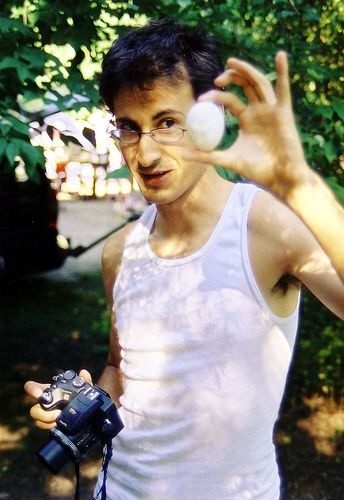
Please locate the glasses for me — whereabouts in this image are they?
[105,112,190,145]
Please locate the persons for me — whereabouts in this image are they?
[23,17,343,500]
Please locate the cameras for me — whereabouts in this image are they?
[37,369,124,476]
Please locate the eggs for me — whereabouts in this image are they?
[185,100,225,150]
